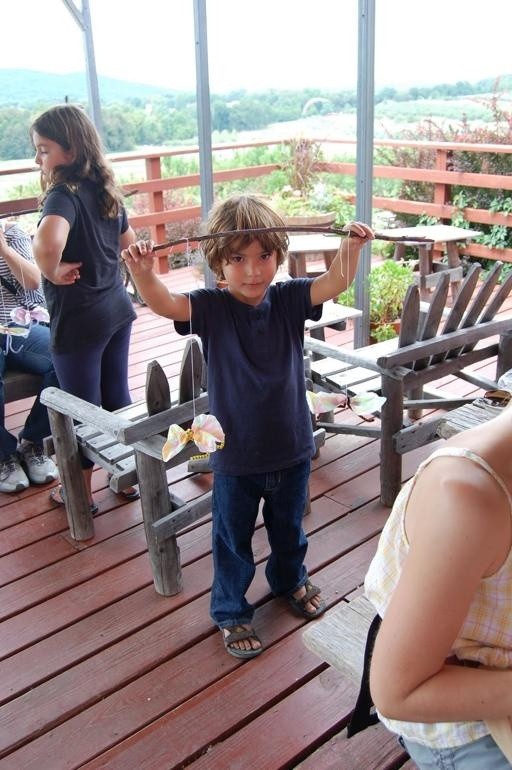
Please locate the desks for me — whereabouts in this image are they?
[376,223,483,307]
[282,232,342,304]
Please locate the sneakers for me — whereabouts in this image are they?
[0,452,30,493]
[17,431,60,486]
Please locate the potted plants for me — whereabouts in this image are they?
[333,259,417,345]
[267,133,350,237]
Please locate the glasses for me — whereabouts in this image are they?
[472,388,512,412]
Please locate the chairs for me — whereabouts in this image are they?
[305,263,512,515]
[42,333,328,597]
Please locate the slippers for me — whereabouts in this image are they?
[107,471,141,501]
[50,485,99,516]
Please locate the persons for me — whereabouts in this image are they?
[358,395,512,770]
[1,218,62,498]
[121,196,374,658]
[28,102,143,514]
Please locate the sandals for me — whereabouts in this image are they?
[283,575,328,619]
[219,617,264,660]
